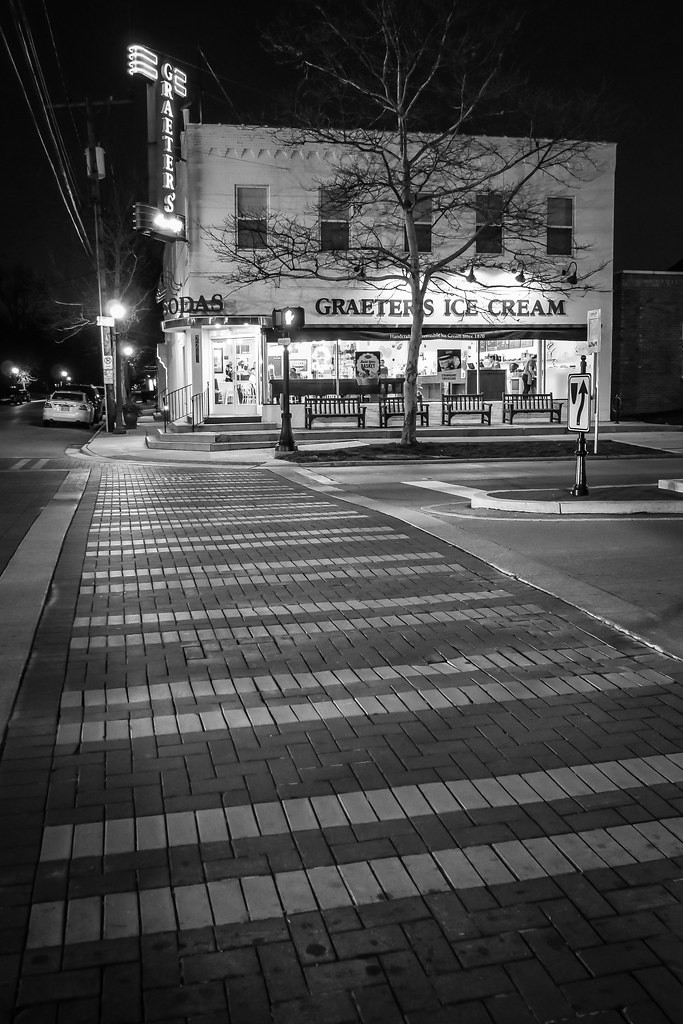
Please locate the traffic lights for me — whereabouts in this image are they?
[272,305,305,331]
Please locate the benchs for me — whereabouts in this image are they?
[378,394,430,428]
[502,392,564,425]
[441,391,493,427]
[305,396,367,430]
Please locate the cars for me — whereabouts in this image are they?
[43,383,105,428]
[0,386,31,405]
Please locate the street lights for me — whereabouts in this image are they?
[105,299,126,435]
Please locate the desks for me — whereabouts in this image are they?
[443,380,465,395]
[269,378,406,403]
[222,382,256,404]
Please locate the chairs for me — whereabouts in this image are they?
[219,383,257,405]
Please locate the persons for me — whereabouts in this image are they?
[522,355,538,400]
[487,356,499,368]
[380,360,388,378]
[290,368,297,378]
[225,365,233,382]
[249,362,257,404]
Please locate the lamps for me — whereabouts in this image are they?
[561,262,578,283]
[354,255,367,278]
[459,262,476,282]
[512,259,525,283]
[156,270,181,303]
